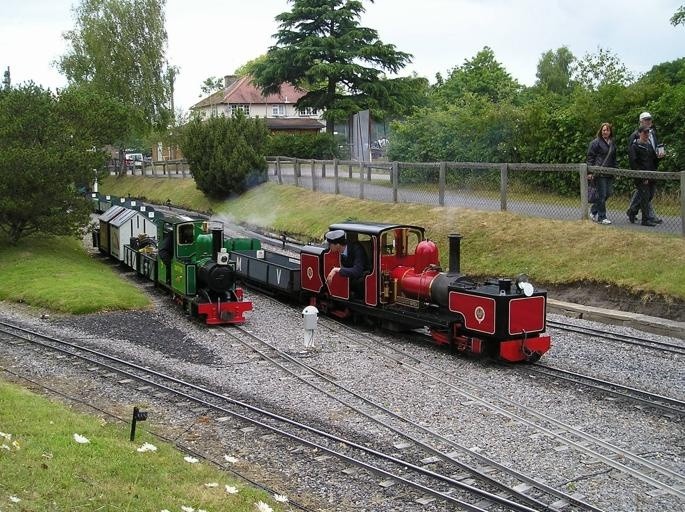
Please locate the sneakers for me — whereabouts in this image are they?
[627,209,663,226]
[598,219,612,225]
[590,211,596,221]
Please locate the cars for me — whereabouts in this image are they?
[126,154,143,169]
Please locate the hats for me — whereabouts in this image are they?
[321,230,345,248]
[640,112,651,118]
[638,126,650,132]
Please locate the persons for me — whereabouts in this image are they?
[629,113,669,224]
[627,124,658,227]
[322,227,373,301]
[586,123,617,224]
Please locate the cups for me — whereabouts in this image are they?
[657,144,665,155]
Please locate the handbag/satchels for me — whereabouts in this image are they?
[588,177,599,203]
